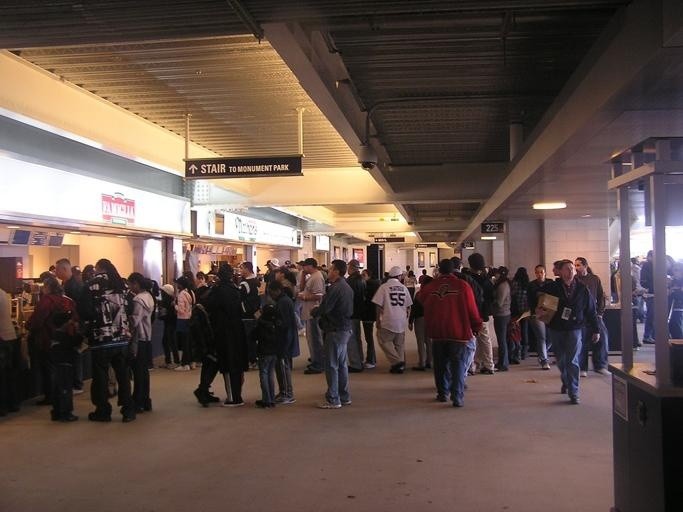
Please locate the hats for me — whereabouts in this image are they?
[241,255,408,278]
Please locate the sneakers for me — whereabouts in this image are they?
[3,384,296,422]
[579,370,588,378]
[450,396,465,408]
[595,367,612,376]
[561,384,568,393]
[316,397,351,408]
[436,393,449,400]
[570,394,580,405]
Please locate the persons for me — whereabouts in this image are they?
[1,249,683,423]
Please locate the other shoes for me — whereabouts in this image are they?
[390,361,405,373]
[542,359,549,369]
[426,365,432,368]
[249,363,260,369]
[304,369,320,374]
[510,359,520,365]
[364,362,375,368]
[480,368,494,374]
[413,366,424,371]
[496,366,509,371]
[348,366,363,373]
[161,361,201,371]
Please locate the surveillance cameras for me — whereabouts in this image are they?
[357,146,378,170]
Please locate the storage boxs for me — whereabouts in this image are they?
[537,293,559,325]
